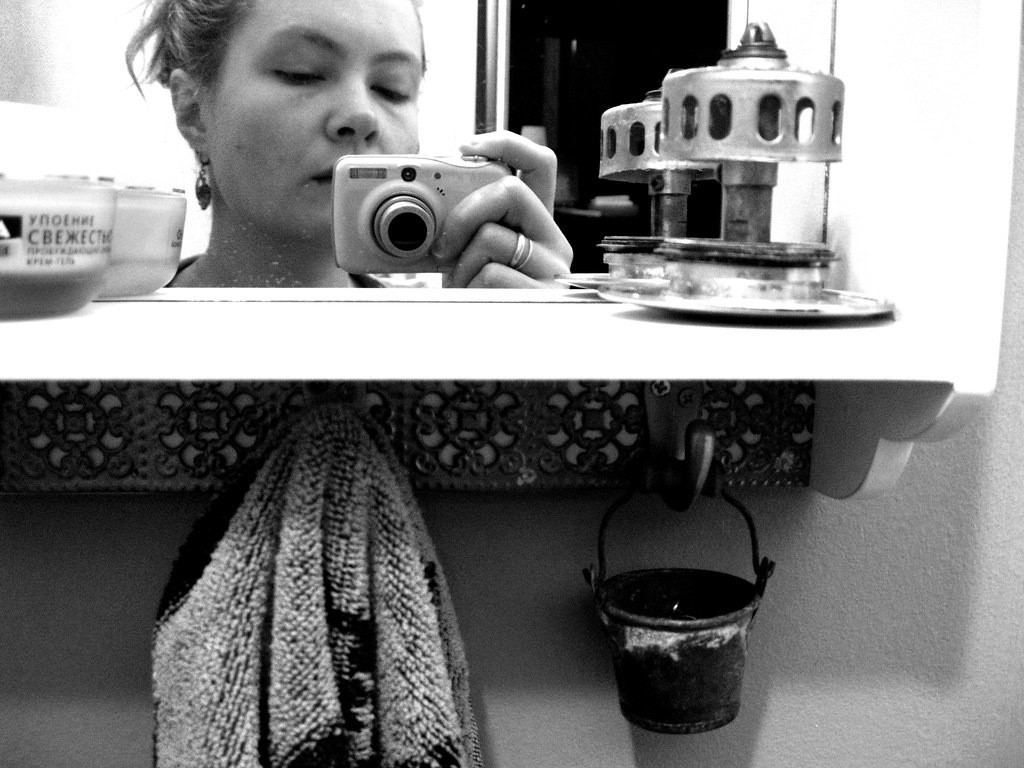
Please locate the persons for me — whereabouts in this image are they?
[125,0,573,288]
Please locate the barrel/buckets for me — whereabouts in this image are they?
[583,487,775,734]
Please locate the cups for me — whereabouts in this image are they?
[0,178,115,317]
[98,189,188,293]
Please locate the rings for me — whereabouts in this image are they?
[510,232,533,269]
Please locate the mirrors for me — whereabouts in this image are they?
[0,0,841,306]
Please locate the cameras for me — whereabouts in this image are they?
[331,154,514,275]
